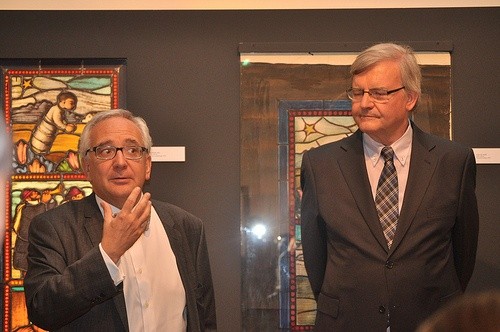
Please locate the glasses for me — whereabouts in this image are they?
[84,145,149,161]
[346,86,405,102]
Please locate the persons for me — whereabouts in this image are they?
[22,109,218,332]
[300,43,478,331]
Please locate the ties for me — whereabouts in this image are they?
[375,146,400,251]
